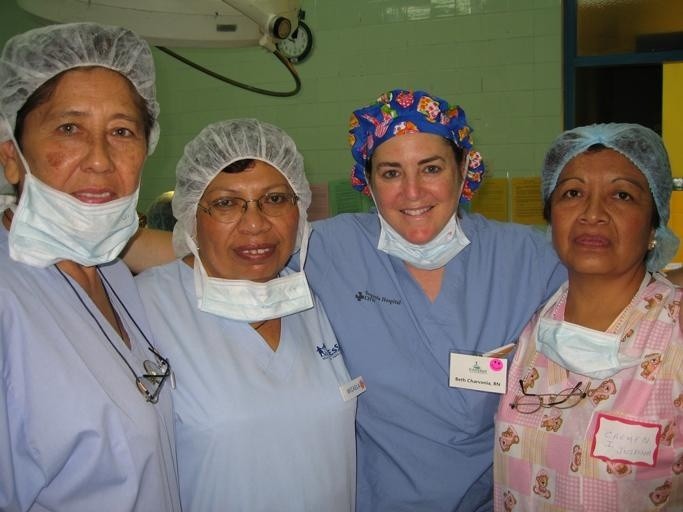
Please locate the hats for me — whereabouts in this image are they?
[348,90,484,204]
[541,123,679,273]
[0,22,161,156]
[171,118,312,258]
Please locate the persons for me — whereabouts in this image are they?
[491,123,682,512]
[116,89,568,511]
[132,117,365,511]
[1,20,179,512]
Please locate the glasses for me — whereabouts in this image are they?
[136,347,170,403]
[198,192,299,223]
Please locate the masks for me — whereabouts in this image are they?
[535,317,620,380]
[8,122,139,267]
[183,226,313,324]
[363,155,469,270]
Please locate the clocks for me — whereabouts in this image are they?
[275,20,312,62]
